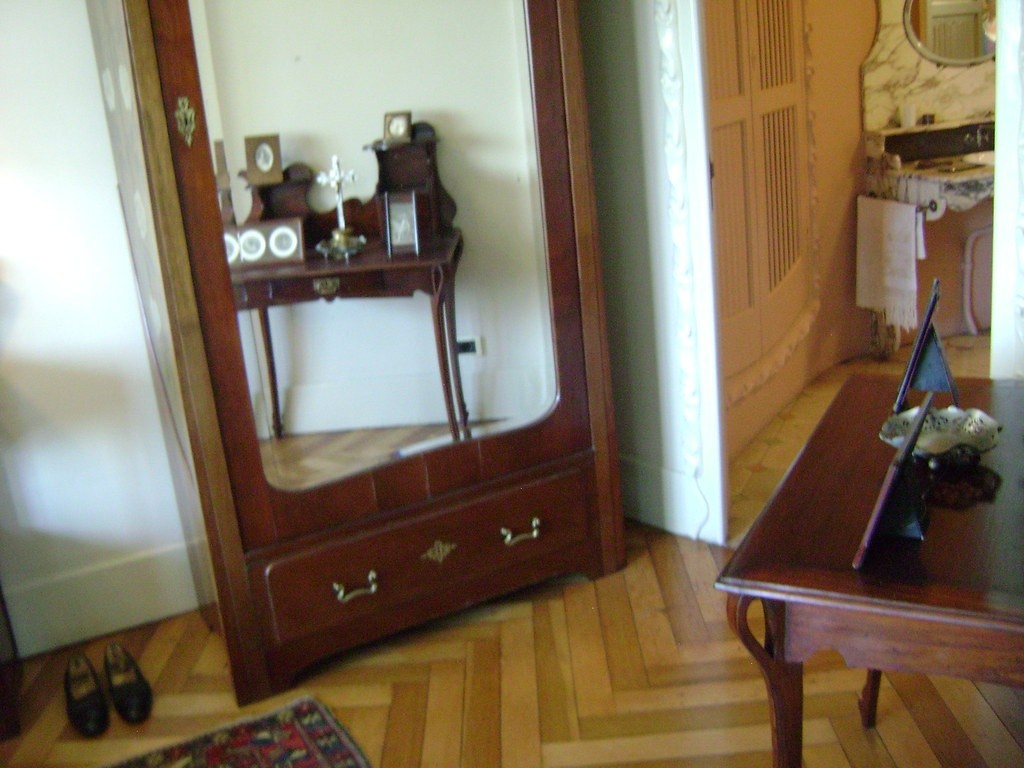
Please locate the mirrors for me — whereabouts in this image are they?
[903,1,997,68]
[176,1,563,491]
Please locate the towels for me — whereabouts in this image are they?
[856,194,926,334]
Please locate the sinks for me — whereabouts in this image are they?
[962,150,994,168]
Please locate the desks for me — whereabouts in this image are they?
[715,371,1024,767]
[226,230,474,457]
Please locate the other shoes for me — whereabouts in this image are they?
[63,652,108,737]
[103,644,152,725]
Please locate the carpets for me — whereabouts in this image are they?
[108,693,368,767]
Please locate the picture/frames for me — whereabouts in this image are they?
[382,110,412,144]
[245,134,283,187]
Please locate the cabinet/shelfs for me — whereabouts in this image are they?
[83,0,629,707]
